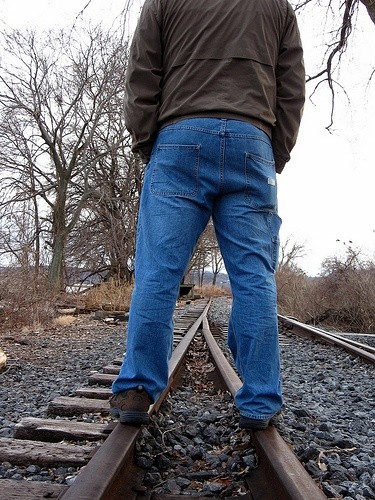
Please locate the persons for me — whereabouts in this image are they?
[105,0,305,432]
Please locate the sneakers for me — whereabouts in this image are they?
[109,387,154,424]
[239,413,272,430]
